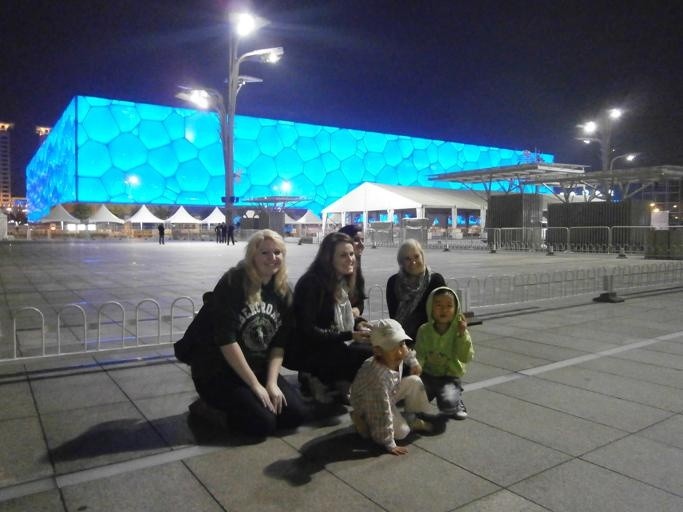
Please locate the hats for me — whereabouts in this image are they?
[370,319,413,351]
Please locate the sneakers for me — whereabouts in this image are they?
[455,400,467,419]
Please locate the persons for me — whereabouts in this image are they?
[227,222,234,246]
[387,239,447,376]
[338,225,373,333]
[158,223,164,245]
[349,319,434,456]
[412,286,474,420]
[221,222,227,243]
[173,229,308,436]
[281,233,374,405]
[215,223,221,243]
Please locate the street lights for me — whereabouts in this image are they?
[179,10,284,241]
[574,103,638,204]
